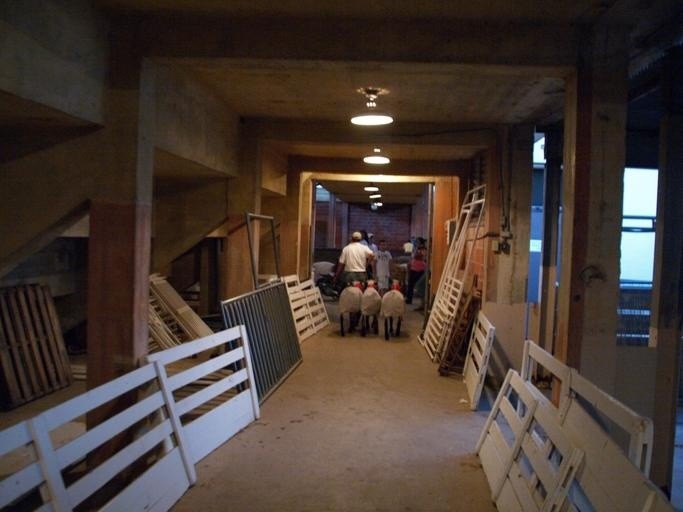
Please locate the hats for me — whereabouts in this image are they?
[352,231,362,240]
[368,233,374,238]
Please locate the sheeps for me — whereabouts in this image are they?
[338,279,406,340]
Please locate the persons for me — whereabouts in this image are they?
[313,228,433,305]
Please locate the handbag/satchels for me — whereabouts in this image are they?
[410,259,426,272]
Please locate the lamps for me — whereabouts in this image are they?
[349,88,396,128]
[362,137,391,166]
[363,183,384,207]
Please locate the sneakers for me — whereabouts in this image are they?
[405,299,412,303]
